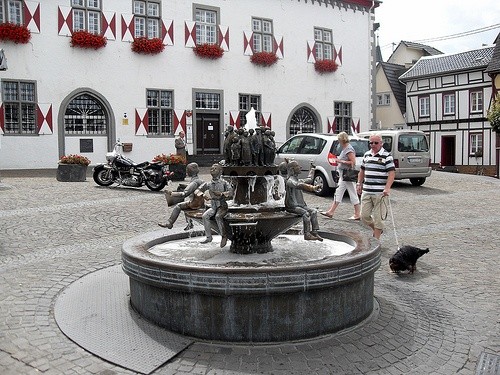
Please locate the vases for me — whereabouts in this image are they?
[57,164,89,182]
[167,165,186,181]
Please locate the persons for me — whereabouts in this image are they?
[355,133,396,242]
[160,161,324,248]
[319,132,363,221]
[224,125,277,167]
[175,130,186,158]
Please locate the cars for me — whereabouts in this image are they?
[273,133,370,197]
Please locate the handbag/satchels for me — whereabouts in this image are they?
[343,164,360,181]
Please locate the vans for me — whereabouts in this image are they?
[356,129,433,186]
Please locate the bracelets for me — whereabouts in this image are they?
[356,183,361,185]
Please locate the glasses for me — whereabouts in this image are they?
[370,142,380,144]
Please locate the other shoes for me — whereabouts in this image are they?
[320,211,333,218]
[348,217,360,220]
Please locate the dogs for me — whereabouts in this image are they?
[388,243,430,277]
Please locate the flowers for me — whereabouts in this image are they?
[60,154,91,164]
[152,153,186,164]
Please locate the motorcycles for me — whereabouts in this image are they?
[93,137,168,192]
